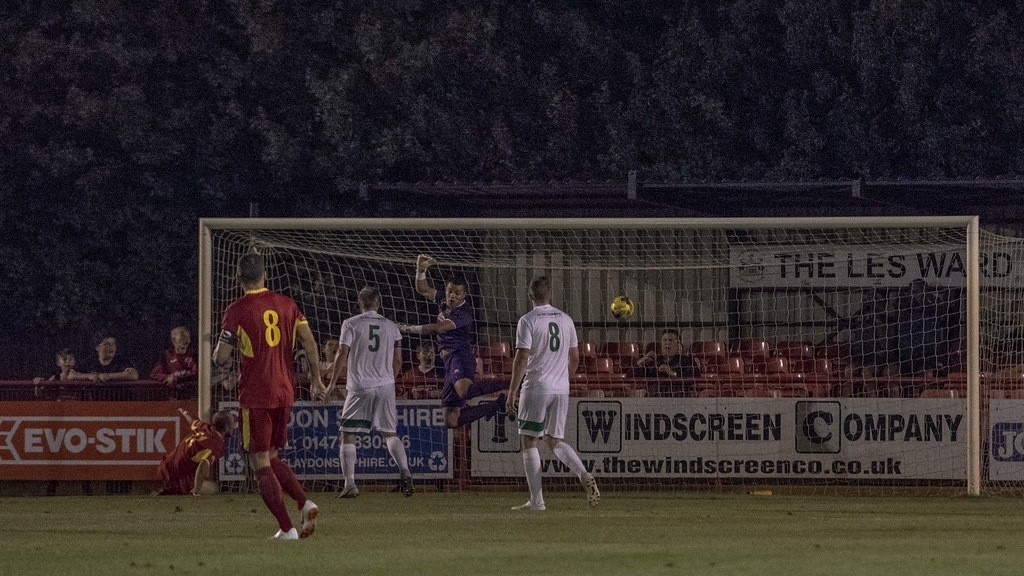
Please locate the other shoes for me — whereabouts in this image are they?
[498,392,516,422]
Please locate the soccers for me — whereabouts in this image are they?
[611,296,634,320]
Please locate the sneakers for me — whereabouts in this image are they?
[298,499,319,539]
[510,501,546,512]
[271,527,298,540]
[581,472,600,507]
[337,483,360,499]
[401,469,415,497]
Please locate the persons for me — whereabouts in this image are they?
[153,408,235,496]
[853,279,967,397]
[34,320,454,495]
[504,277,601,510]
[398,253,523,428]
[327,286,416,498]
[637,329,701,397]
[212,252,327,540]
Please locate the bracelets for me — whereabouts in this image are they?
[415,267,427,281]
[673,370,678,378]
[192,490,200,496]
[183,409,192,417]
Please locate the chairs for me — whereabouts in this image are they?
[473,341,1024,398]
